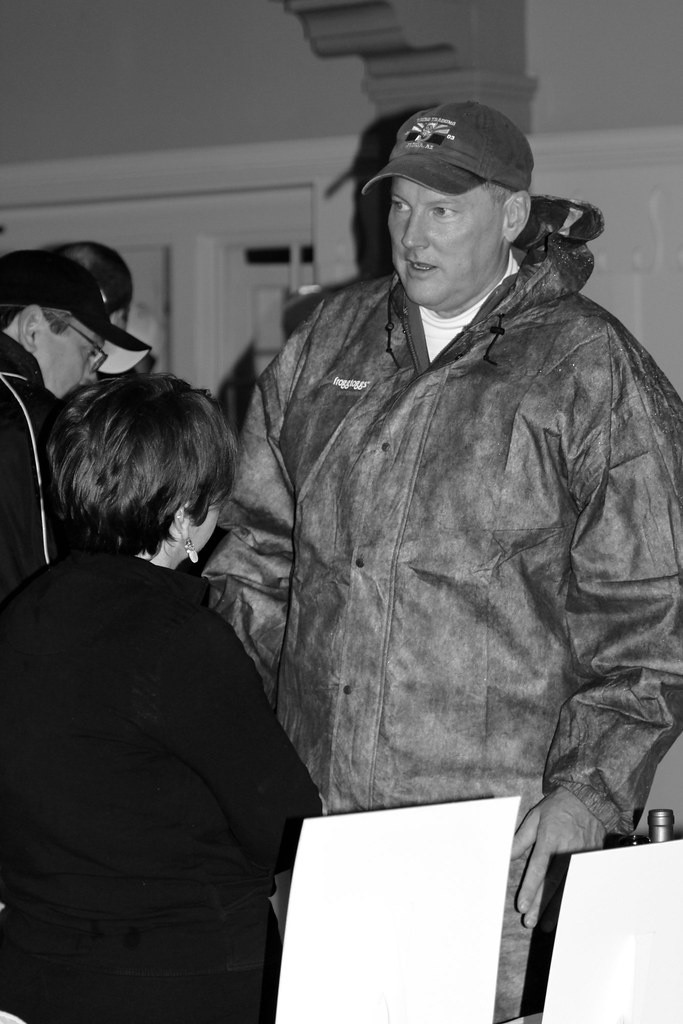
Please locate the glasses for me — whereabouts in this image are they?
[47,310,113,374]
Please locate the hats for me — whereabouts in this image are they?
[361,102,536,204]
[0,248,154,377]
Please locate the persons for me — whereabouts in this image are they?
[55,241,131,332]
[0,250,144,597]
[2,377,323,1024]
[203,104,682,1024]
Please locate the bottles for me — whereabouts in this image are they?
[648,809,675,842]
[617,834,647,847]
